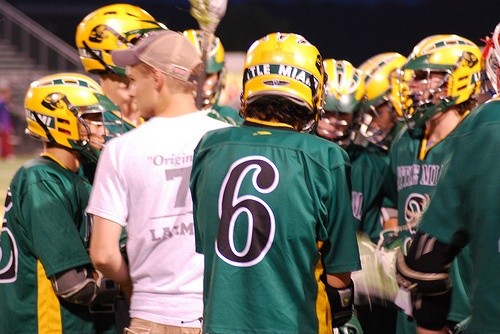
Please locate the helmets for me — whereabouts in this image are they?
[316,56,367,116]
[236,30,329,134]
[23,71,108,152]
[401,33,483,107]
[73,2,168,79]
[355,50,417,121]
[179,27,225,111]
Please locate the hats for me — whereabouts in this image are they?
[111,29,204,84]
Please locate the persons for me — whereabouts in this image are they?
[76,4,174,262]
[84,30,234,334]
[304,34,482,334]
[395,23,500,334]
[0,79,17,160]
[0,72,130,334]
[182,29,244,126]
[188,32,362,334]
